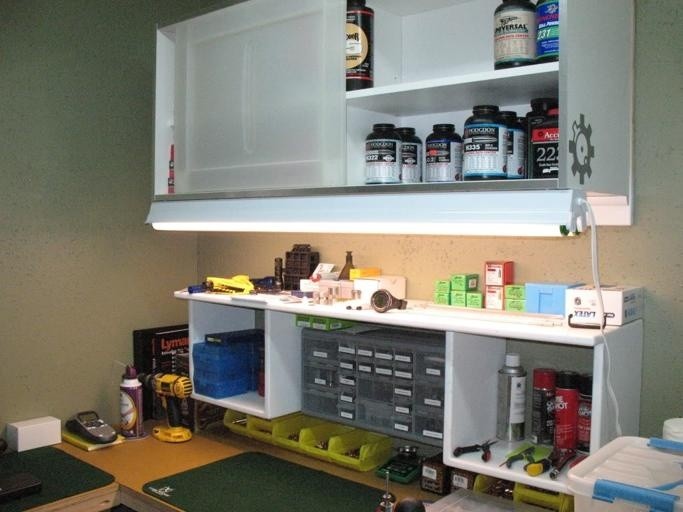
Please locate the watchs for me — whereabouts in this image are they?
[369,288,407,313]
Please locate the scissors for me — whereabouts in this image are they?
[454,439,498,461]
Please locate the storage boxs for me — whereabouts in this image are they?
[566,436,683,511]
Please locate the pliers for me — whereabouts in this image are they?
[506,446,535,471]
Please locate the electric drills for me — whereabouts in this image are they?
[135,372,193,443]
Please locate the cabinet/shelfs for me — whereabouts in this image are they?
[173,284,644,496]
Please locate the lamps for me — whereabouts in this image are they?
[145,190,587,237]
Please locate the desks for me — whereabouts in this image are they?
[0,420,451,512]
